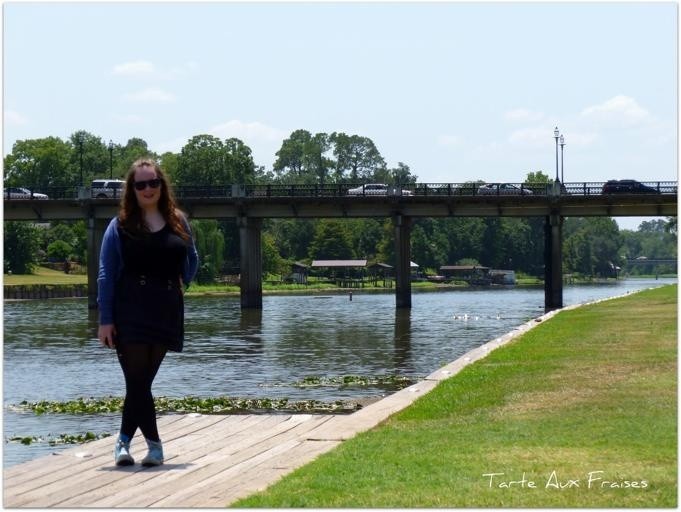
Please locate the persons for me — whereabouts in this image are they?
[95,156,201,471]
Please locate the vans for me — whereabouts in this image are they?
[89,179,131,199]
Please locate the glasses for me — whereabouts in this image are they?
[131,178,162,191]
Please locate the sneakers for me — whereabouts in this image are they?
[113,435,134,468]
[139,439,165,468]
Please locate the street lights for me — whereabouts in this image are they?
[551,125,566,196]
[107,139,115,179]
[77,134,84,189]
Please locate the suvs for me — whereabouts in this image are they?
[599,178,663,193]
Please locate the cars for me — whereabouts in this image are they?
[477,182,534,195]
[345,185,415,196]
[635,256,647,261]
[3,187,49,199]
